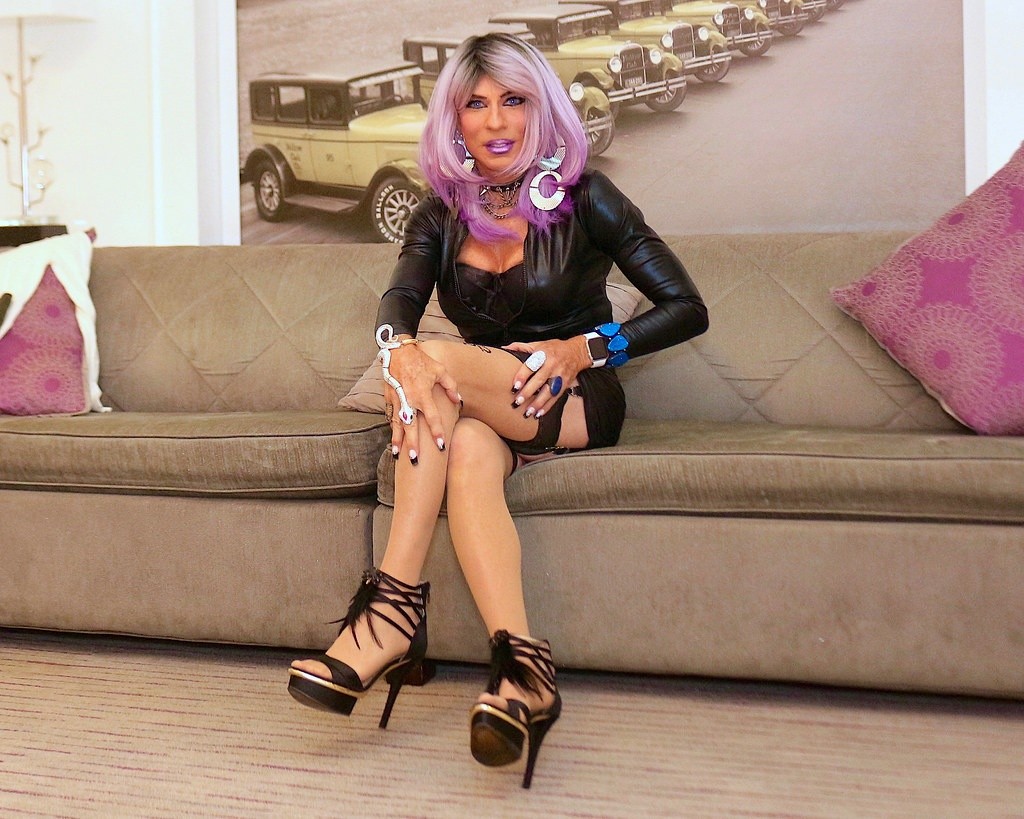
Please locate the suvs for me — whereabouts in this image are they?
[238,56,450,249]
[400,0,848,162]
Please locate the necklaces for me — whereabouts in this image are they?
[472,159,526,220]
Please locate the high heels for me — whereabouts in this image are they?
[469,629,562,790]
[287,566,431,730]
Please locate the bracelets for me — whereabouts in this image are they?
[376,323,419,425]
[593,322,631,368]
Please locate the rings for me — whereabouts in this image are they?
[384,402,393,423]
[526,349,547,373]
[547,376,564,396]
[410,407,418,415]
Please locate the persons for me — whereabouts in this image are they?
[289,30,711,789]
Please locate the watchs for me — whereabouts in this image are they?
[583,332,610,368]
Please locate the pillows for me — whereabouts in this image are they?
[0,229,101,420]
[837,138,1024,440]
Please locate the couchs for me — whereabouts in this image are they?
[0,235,1024,703]
